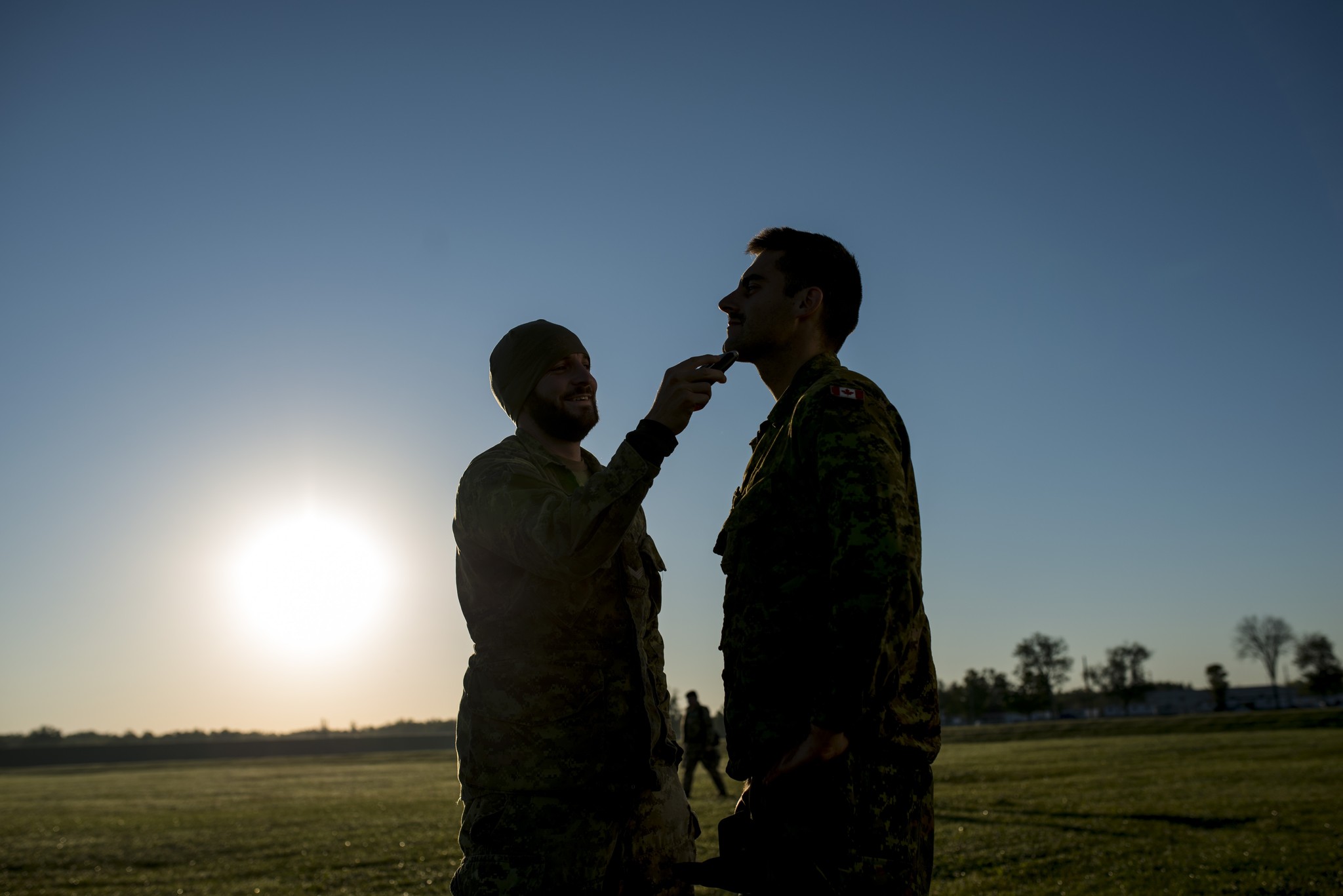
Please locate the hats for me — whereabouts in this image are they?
[489,318,591,428]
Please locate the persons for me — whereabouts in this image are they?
[712,227,943,896]
[452,320,727,896]
[683,691,728,799]
